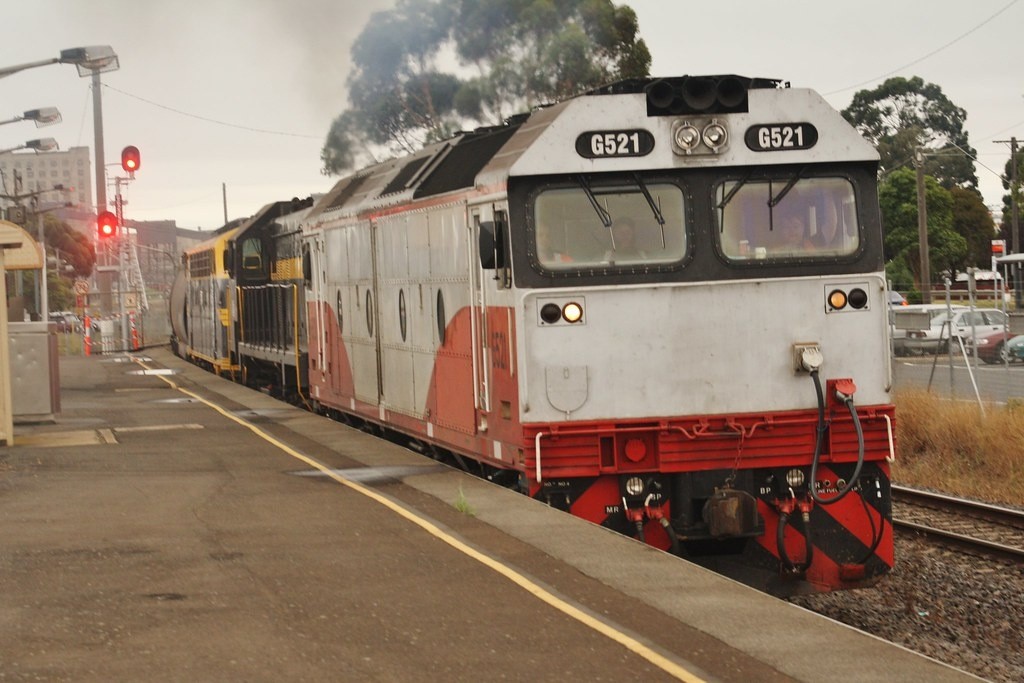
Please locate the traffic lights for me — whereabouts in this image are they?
[121,145,141,171]
[98,213,117,238]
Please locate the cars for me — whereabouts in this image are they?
[967,331,1024,365]
[51,311,85,332]
[905,310,1009,354]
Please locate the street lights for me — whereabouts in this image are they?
[993,239,1010,291]
[14,183,83,316]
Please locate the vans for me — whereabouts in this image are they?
[890,304,982,356]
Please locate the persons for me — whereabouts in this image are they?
[607,216,654,262]
[780,212,814,251]
[535,220,572,263]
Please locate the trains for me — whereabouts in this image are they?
[167,75,897,593]
